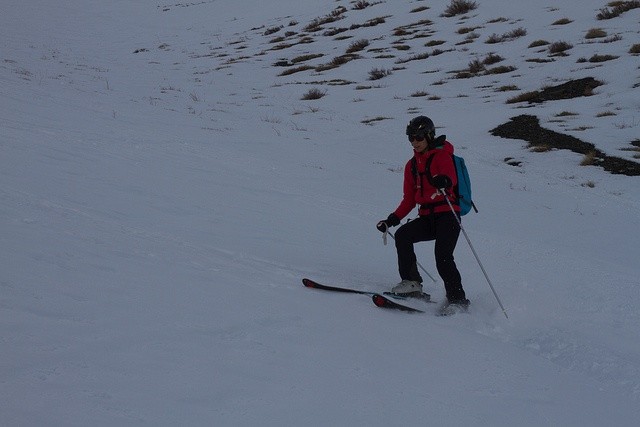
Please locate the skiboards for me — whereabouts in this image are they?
[301,278,439,319]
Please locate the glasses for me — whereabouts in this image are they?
[408,134,425,142]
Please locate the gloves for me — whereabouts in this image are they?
[376,213,400,233]
[432,174,452,190]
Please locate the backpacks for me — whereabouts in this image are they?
[411,155,478,216]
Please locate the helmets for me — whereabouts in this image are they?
[406,116,435,139]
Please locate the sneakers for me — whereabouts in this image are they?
[391,279,423,295]
[439,299,470,316]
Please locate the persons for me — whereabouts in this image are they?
[375,114,471,317]
